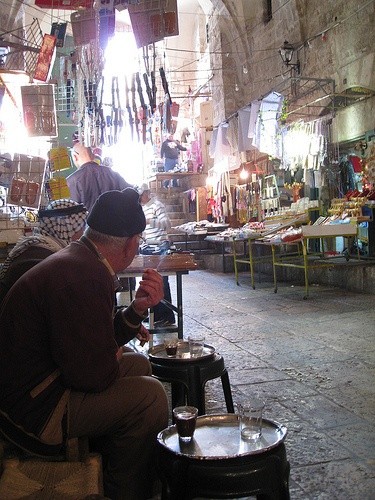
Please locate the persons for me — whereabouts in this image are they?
[137,182,175,326]
[66,143,142,230]
[162,133,187,188]
[0,191,167,500]
[0,198,90,302]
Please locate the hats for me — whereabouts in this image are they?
[137,183,149,196]
[86,186,146,236]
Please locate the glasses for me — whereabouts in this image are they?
[133,234,147,245]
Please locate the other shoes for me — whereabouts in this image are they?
[162,186,168,189]
[173,184,179,187]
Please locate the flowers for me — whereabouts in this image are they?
[283,181,303,190]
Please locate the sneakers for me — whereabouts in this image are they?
[153,320,172,327]
[144,316,149,322]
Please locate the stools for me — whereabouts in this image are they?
[149,353,234,416]
[157,442,290,500]
[0,451,104,500]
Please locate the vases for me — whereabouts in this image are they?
[293,189,299,196]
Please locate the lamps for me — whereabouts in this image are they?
[279,39,300,75]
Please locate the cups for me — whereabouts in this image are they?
[237,398,265,441]
[164,337,179,358]
[173,406,199,442]
[188,335,205,357]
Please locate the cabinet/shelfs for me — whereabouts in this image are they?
[116,270,188,339]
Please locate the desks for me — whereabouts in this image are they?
[147,172,200,192]
